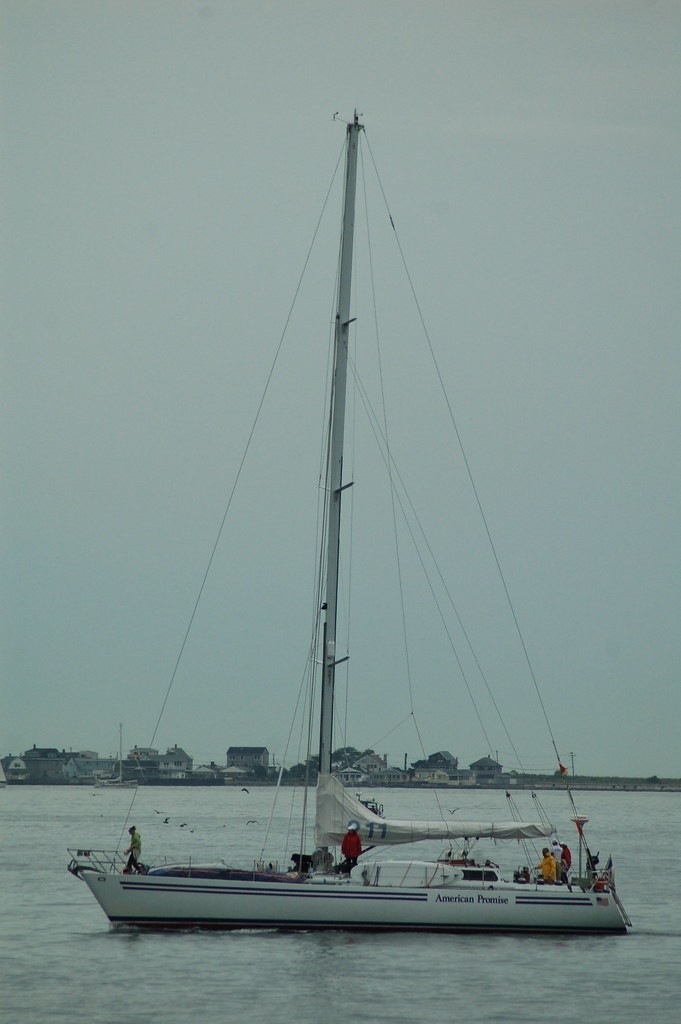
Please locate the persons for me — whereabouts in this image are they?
[550,840,571,885]
[534,848,556,883]
[123,825,142,875]
[342,825,362,875]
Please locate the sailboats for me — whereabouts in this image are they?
[94,722,141,788]
[65,107,633,935]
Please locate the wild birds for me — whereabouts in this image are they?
[447,807,460,815]
[87,792,102,797]
[151,787,259,834]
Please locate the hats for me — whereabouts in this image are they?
[347,826,355,829]
[552,840,558,843]
